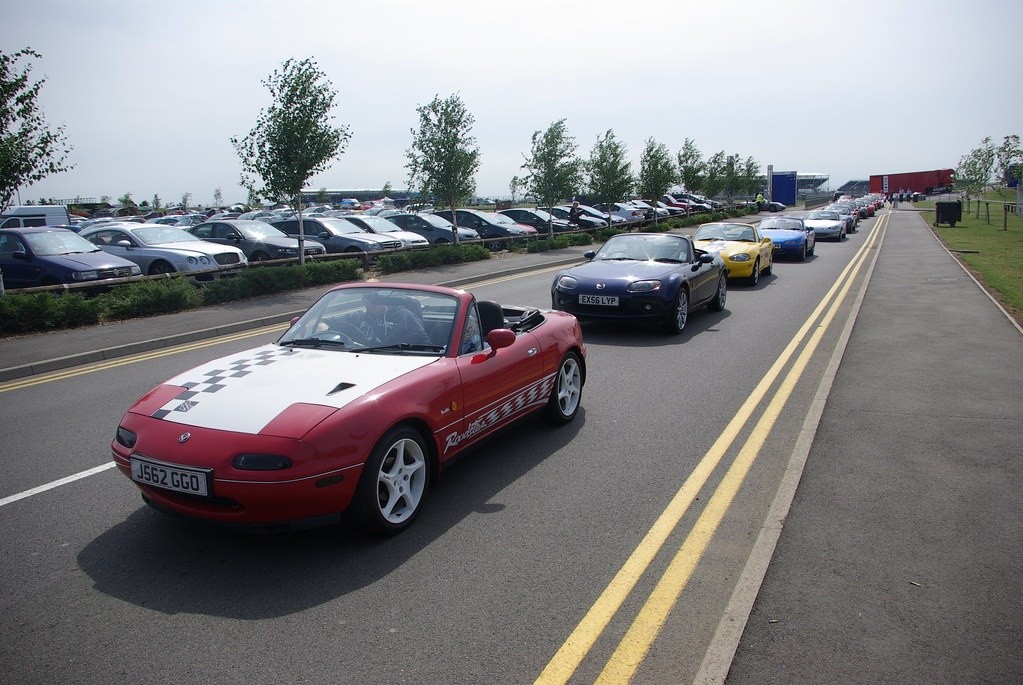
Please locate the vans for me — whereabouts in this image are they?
[0,205,71,228]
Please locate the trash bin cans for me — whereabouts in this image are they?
[933,200,963,228]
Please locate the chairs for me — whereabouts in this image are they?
[382,294,424,323]
[626,240,649,260]
[466,300,505,354]
[680,239,696,264]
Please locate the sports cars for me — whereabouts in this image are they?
[109,280,586,534]
[825,192,926,233]
[801,209,847,242]
[685,222,774,287]
[550,233,729,335]
[755,217,816,261]
[741,199,786,213]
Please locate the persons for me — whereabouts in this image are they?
[898,187,904,202]
[441,315,491,354]
[754,191,763,212]
[289,278,433,352]
[906,188,911,202]
[569,201,583,225]
[889,189,894,203]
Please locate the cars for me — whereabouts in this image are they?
[269,218,401,264]
[186,220,327,264]
[436,207,524,251]
[338,216,430,250]
[70,198,436,230]
[487,192,722,240]
[79,225,250,279]
[384,214,480,244]
[2,228,141,295]
